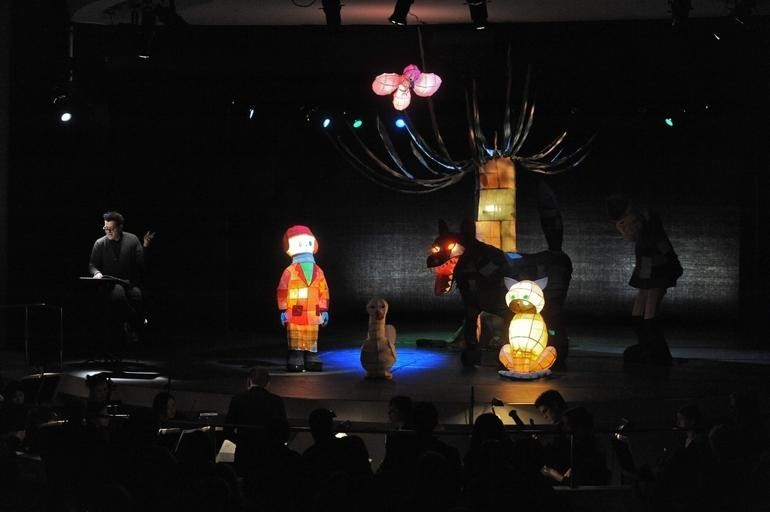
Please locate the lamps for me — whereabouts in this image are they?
[48,94,335,131]
[389,0,489,30]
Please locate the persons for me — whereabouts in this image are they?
[605,194,684,373]
[534,391,607,485]
[152,393,242,498]
[223,368,288,510]
[676,392,769,511]
[303,409,371,510]
[3,381,43,481]
[88,209,158,360]
[387,395,517,510]
[89,379,131,484]
